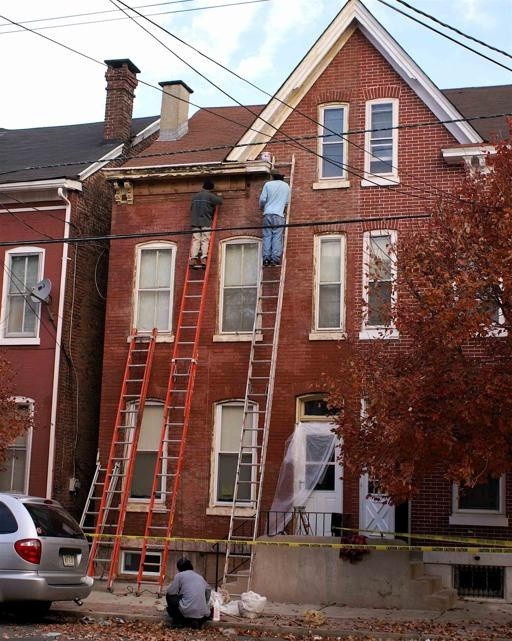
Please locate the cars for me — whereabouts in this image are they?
[0,492,95,622]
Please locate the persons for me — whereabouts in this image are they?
[164,557,215,632]
[188,181,223,272]
[258,170,291,268]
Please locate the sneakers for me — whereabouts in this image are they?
[264,260,277,266]
[188,258,207,266]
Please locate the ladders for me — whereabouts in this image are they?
[80,326,160,594]
[222,153,295,596]
[134,196,222,597]
